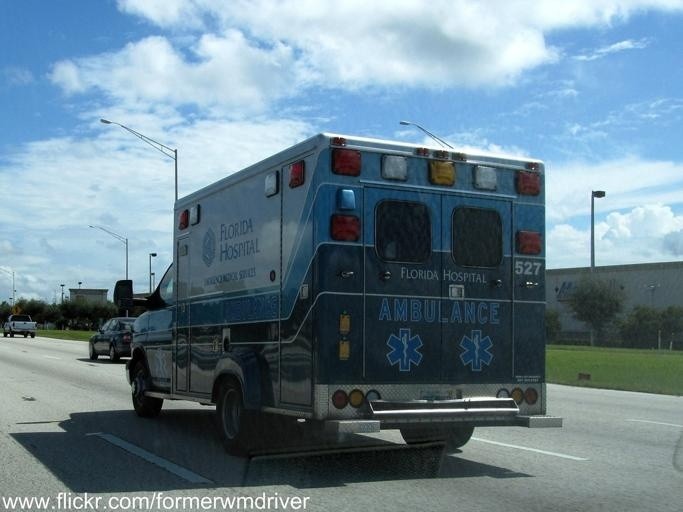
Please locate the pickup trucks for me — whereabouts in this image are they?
[3,314,37,338]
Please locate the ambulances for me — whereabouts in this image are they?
[114,132,563,456]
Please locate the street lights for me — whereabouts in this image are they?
[60,284,65,304]
[78,281,82,289]
[399,120,454,149]
[87,224,128,317]
[589,190,605,347]
[149,253,157,294]
[100,118,178,199]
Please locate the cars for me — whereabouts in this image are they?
[89,317,138,362]
